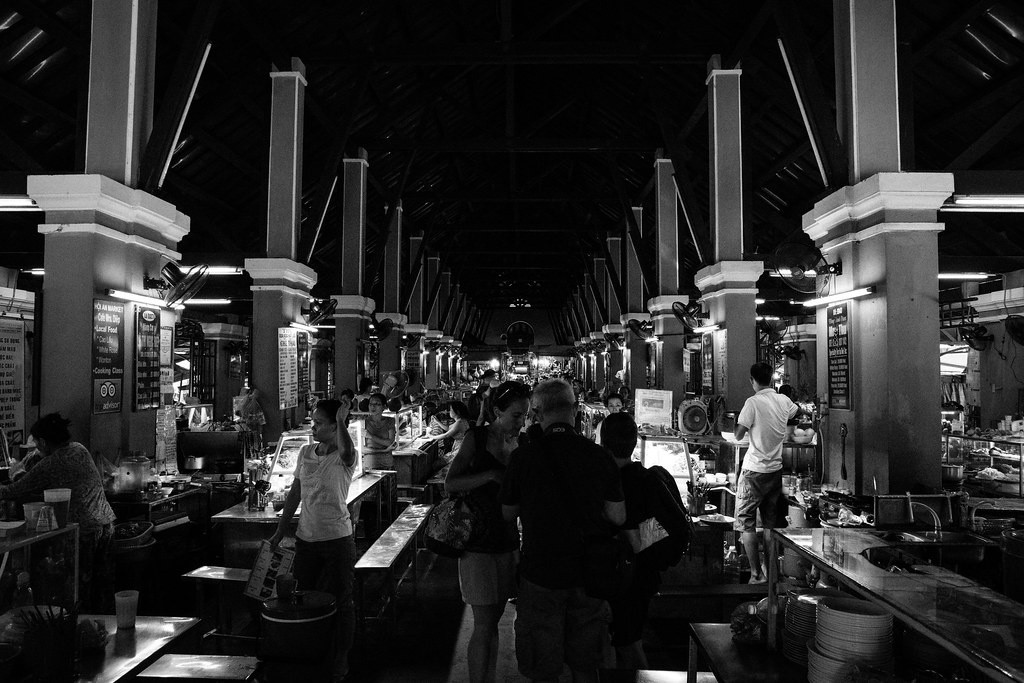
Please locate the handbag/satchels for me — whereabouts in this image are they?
[422,497,472,558]
[659,553,719,587]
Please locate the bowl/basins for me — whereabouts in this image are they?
[793,437,813,443]
[942,465,963,480]
[161,487,174,497]
[721,432,749,444]
[162,480,186,492]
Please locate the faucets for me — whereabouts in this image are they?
[909,501,942,541]
[970,500,995,533]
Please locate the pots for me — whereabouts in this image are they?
[184,455,205,469]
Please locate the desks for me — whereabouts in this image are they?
[582,402,1024,683]
[110,437,439,562]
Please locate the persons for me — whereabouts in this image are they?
[735,363,802,584]
[0,411,116,614]
[268,367,693,683]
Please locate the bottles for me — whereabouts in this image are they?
[782,472,804,496]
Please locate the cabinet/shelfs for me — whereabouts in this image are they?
[942,432,1024,500]
[0,520,81,615]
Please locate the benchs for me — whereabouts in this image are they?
[396,463,452,506]
[135,653,257,683]
[351,503,435,619]
[687,621,786,683]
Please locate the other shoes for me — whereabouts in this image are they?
[749,574,766,585]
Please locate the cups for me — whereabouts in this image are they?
[115,590,139,628]
[36,506,58,532]
[23,501,45,528]
[43,486,71,527]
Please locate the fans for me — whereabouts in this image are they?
[425,341,468,359]
[960,326,994,351]
[143,263,210,309]
[773,243,842,294]
[360,318,393,342]
[1005,315,1024,345]
[401,332,421,348]
[628,319,655,340]
[757,318,788,349]
[678,399,707,435]
[567,333,625,356]
[784,346,806,360]
[672,300,710,330]
[301,299,338,326]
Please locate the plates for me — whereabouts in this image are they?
[782,588,893,683]
[698,515,736,523]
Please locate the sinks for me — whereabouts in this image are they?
[913,530,995,546]
[867,529,935,547]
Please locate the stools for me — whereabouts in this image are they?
[183,565,259,642]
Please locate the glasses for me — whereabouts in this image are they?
[497,384,531,400]
[368,403,382,407]
